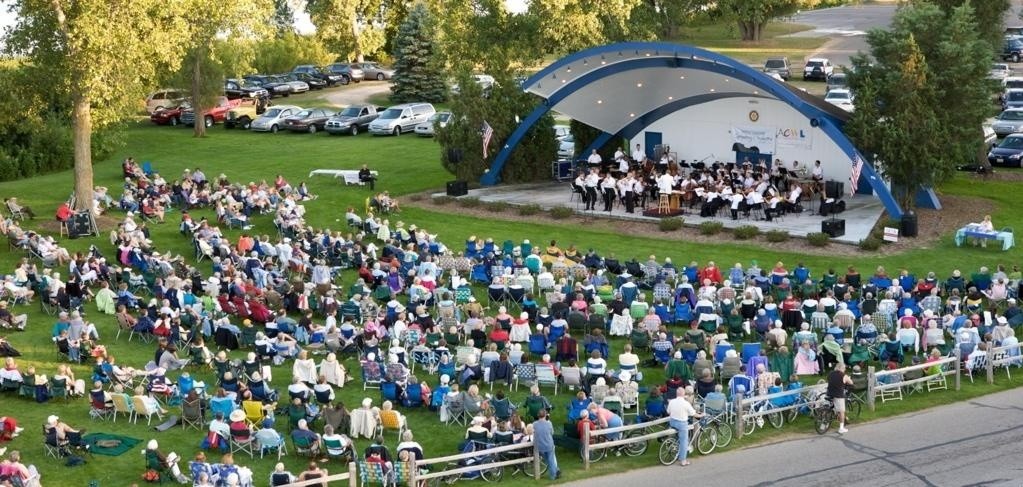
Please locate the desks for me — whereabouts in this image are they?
[955,224,1015,252]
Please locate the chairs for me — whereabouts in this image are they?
[652,267,1021,430]
[424,234,658,487]
[0,171,453,487]
[568,170,827,223]
[998,226,1014,249]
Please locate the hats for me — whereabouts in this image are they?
[1,215,1017,485]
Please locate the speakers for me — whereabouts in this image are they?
[448,149,464,163]
[825,181,844,199]
[446,179,468,197]
[822,218,846,238]
[902,214,917,236]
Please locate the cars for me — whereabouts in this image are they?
[246,102,303,135]
[555,132,574,161]
[282,107,336,133]
[409,110,453,137]
[142,57,394,128]
[956,24,1023,172]
[447,69,532,102]
[822,86,854,113]
[550,123,571,141]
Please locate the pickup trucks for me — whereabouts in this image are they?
[321,102,385,136]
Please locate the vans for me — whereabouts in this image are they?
[365,100,437,138]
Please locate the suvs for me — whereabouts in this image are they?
[762,55,792,81]
[824,72,853,97]
[802,57,833,81]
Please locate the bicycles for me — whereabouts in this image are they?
[811,383,860,437]
[783,389,827,426]
[478,443,549,483]
[740,385,785,437]
[572,409,734,467]
